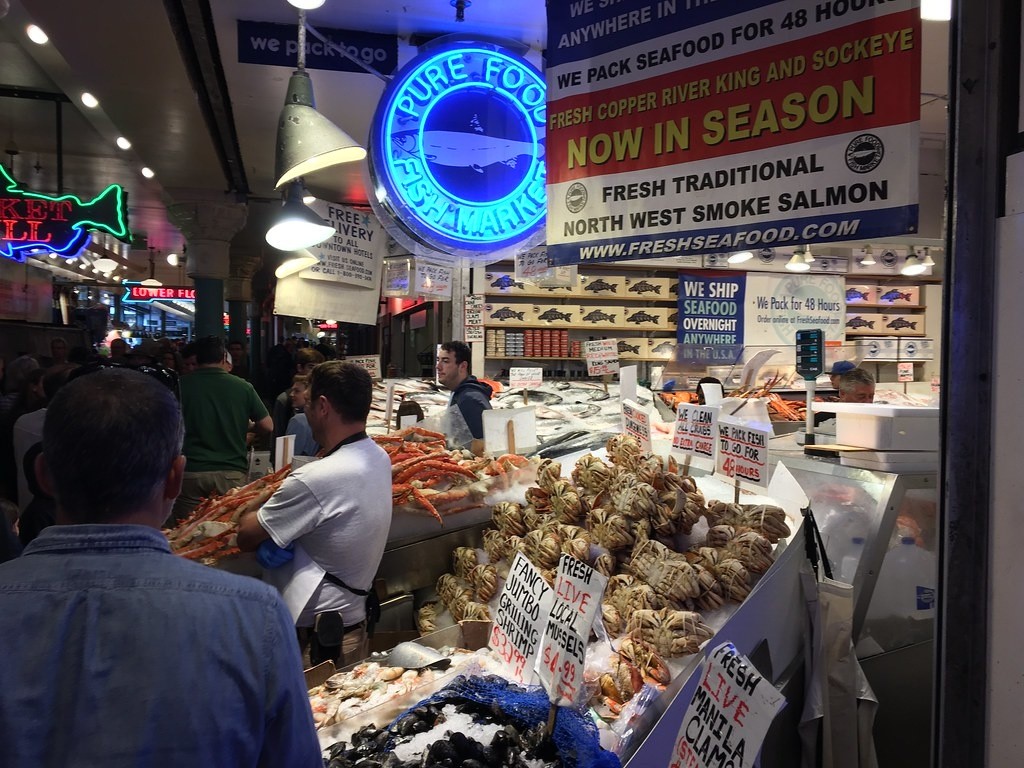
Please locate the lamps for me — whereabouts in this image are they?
[265,187,336,251]
[901,245,926,276]
[92,231,119,272]
[804,244,815,262]
[271,248,320,279]
[785,245,810,271]
[274,0,367,188]
[860,244,876,264]
[922,247,935,266]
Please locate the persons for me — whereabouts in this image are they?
[824,360,856,389]
[0,368,324,768]
[236,361,393,670]
[174,336,274,512]
[96,338,199,390]
[223,335,336,456]
[435,340,492,458]
[0,353,96,564]
[696,377,724,405]
[839,368,875,403]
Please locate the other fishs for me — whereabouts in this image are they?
[365,376,651,462]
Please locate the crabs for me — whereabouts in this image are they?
[158,427,532,562]
[419,433,791,733]
[658,368,833,423]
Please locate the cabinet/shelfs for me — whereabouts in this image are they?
[483,258,942,381]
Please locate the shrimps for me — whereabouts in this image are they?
[308,645,505,734]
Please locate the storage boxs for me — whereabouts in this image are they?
[836,412,940,450]
[854,336,898,362]
[899,337,934,361]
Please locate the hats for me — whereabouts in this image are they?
[824,360,856,375]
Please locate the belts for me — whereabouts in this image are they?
[344,621,364,635]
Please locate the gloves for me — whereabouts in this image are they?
[253,537,295,571]
[259,502,264,508]
